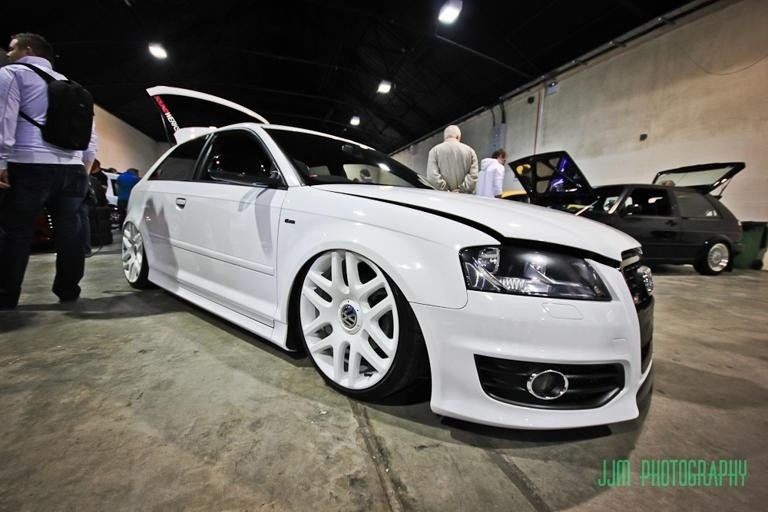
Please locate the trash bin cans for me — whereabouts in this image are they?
[733,221,768,270]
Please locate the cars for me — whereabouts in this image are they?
[119,85,655,431]
[508,150,746,276]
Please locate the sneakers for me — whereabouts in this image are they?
[60,285,81,304]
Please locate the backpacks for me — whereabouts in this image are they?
[11,62,94,151]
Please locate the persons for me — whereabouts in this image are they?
[79,160,140,257]
[475,149,506,198]
[427,125,479,195]
[359,169,375,183]
[0,33,97,310]
